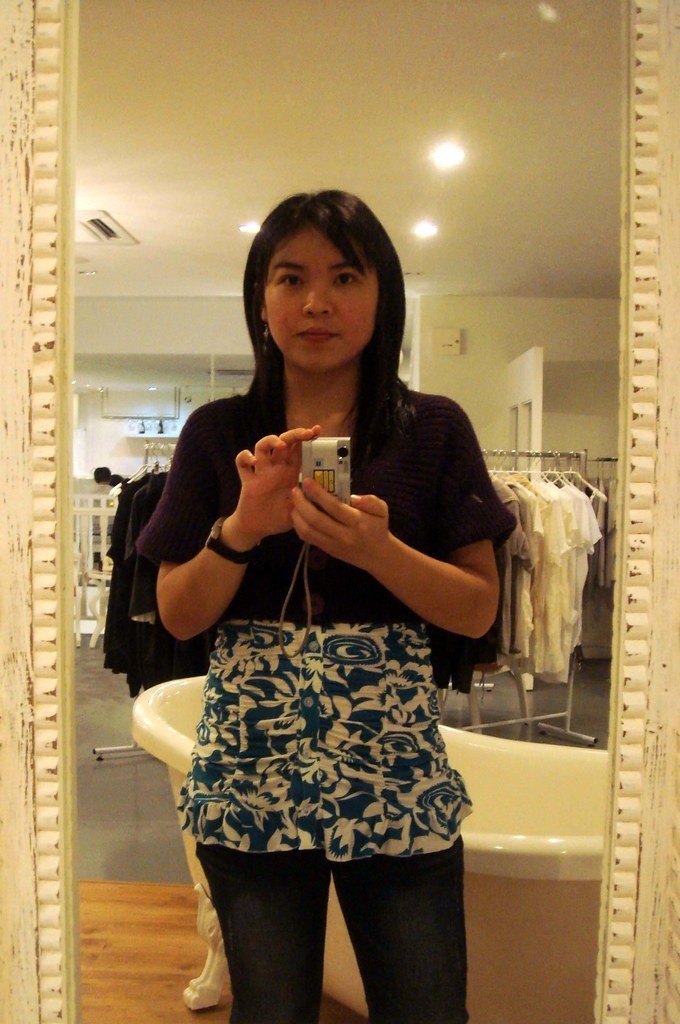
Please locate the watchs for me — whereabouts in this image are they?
[206,517,252,565]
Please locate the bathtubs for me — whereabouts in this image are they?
[127,667,608,1024]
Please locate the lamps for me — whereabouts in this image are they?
[156,419,164,435]
[139,418,146,435]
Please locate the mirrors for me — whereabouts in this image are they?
[1,3,680,1023]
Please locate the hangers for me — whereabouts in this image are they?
[107,438,181,497]
[483,447,621,500]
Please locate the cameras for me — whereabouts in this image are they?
[303,437,351,512]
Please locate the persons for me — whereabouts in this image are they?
[134,189,518,1024]
[92,467,123,570]
